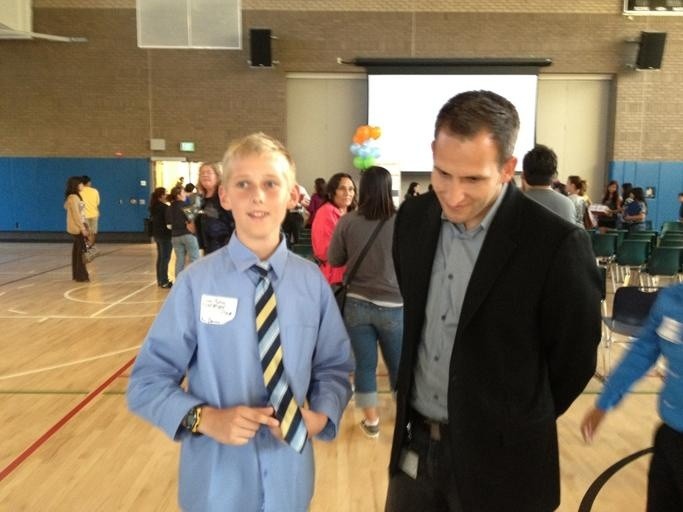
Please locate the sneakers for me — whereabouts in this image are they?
[158,280,173,288]
[359,420,380,438]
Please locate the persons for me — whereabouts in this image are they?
[80,174,100,249]
[63,176,91,283]
[382,89,602,511]
[126,129,357,512]
[327,166,403,437]
[580,282,682,511]
[145,141,683,312]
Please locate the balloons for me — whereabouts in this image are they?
[350,125,381,172]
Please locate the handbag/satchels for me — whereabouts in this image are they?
[583,207,597,229]
[330,282,348,313]
[82,246,96,263]
[144,218,153,236]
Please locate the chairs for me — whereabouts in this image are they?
[582,220,683,395]
[291,227,312,258]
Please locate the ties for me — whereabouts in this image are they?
[249,261,309,455]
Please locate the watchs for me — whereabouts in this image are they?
[184,406,202,436]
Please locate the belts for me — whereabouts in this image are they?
[416,416,449,442]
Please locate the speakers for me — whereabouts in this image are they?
[249,27,273,67]
[636,31,666,69]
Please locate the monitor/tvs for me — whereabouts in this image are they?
[623,0,683,17]
